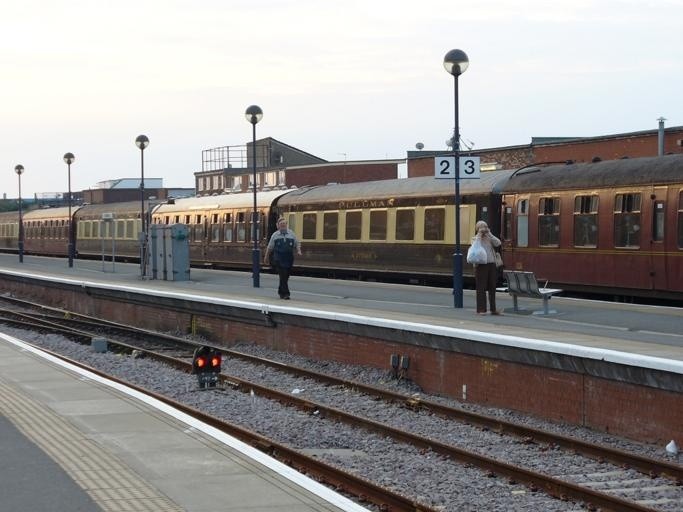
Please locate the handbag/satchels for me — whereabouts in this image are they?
[494,253,503,268]
[467,240,486,264]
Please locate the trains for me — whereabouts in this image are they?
[0,153,683,304]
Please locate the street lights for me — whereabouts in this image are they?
[245,105,263,287]
[63,152,74,266]
[15,165,24,263]
[443,49,469,307]
[135,136,149,275]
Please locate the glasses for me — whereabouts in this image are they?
[279,222,286,225]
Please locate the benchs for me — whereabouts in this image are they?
[502,270,563,316]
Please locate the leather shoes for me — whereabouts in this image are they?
[280,295,290,300]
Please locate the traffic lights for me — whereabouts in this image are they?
[192,343,221,388]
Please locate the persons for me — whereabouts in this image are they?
[264,218,302,299]
[471,220,505,317]
